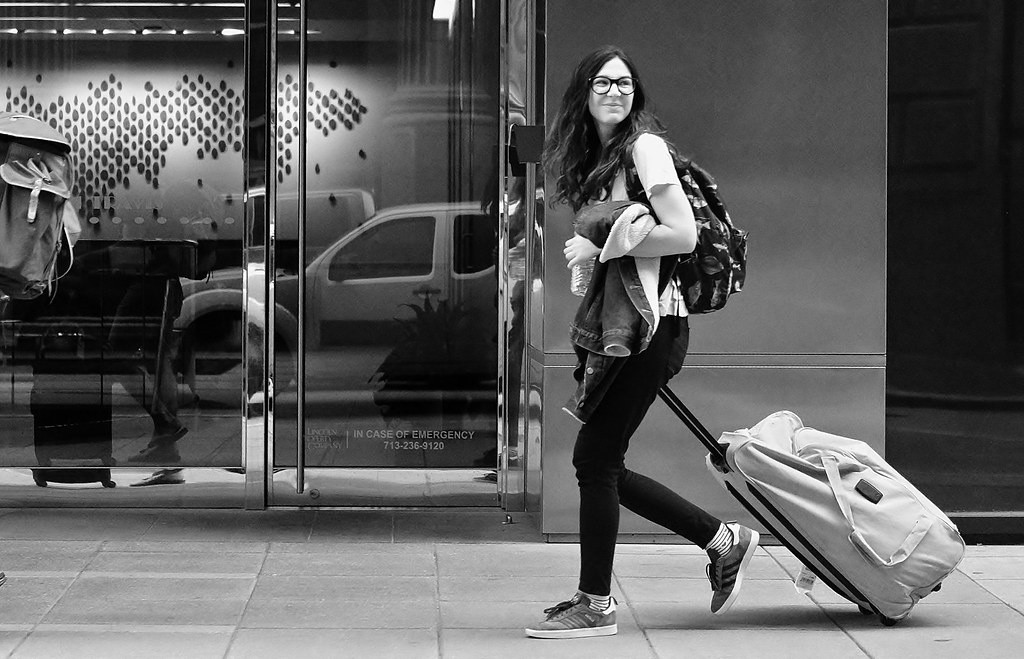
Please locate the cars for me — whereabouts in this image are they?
[167,188,501,404]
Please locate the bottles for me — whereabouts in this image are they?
[571,257,596,296]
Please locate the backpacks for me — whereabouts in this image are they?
[0,112,74,297]
[624,137,749,314]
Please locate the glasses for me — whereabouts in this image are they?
[587,76,638,95]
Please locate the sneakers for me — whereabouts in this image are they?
[525,593,618,639]
[705,518,759,616]
[474,471,498,484]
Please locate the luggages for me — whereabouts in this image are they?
[30,330,118,466]
[659,381,967,624]
[31,468,116,487]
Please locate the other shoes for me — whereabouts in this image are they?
[127,444,181,463]
[148,427,189,447]
[129,472,185,486]
[472,448,519,468]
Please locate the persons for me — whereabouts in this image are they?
[106,274,209,486]
[525,45,759,640]
